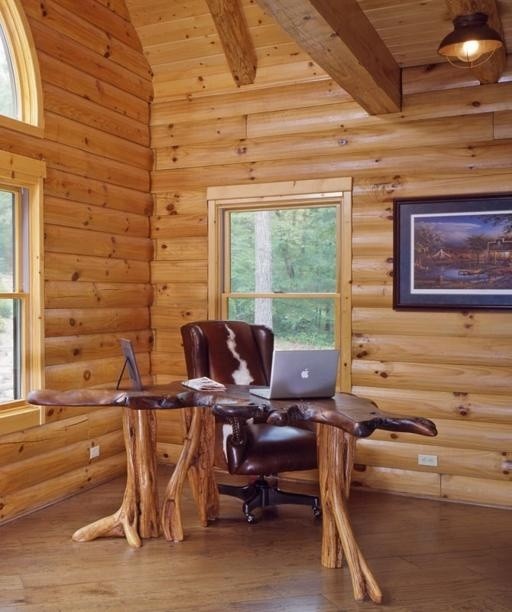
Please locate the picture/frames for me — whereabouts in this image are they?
[120,337,143,391]
[392,190,512,313]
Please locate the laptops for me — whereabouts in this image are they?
[249,349,340,401]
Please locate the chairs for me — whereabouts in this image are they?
[179,318,322,526]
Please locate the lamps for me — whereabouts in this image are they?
[438,3,504,70]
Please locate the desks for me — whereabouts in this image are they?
[25,386,438,612]
